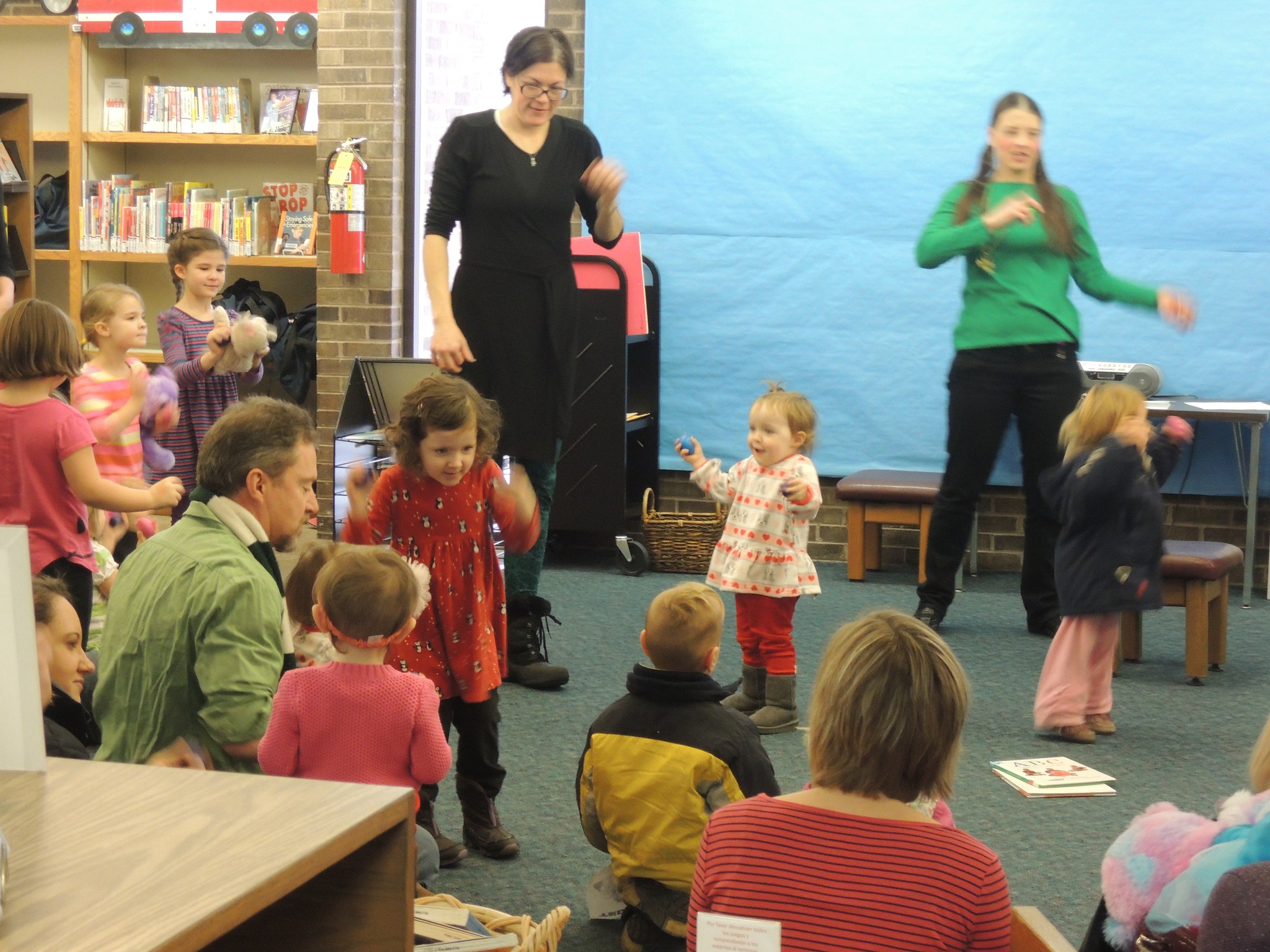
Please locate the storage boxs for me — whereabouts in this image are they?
[411,890,571,952]
[640,487,731,575]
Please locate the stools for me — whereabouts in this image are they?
[1113,539,1245,687]
[834,469,985,593]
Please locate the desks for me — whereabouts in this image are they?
[1134,398,1270,610]
[0,755,425,952]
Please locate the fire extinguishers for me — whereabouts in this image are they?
[323,136,368,274]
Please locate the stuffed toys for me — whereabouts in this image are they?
[1101,788,1261,951]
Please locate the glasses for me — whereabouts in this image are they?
[516,78,569,101]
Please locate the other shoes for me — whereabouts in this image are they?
[1044,724,1097,744]
[1086,713,1117,735]
[1027,614,1063,638]
[913,607,943,632]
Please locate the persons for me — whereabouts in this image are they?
[264,92,283,132]
[0,196,351,781]
[423,27,627,686]
[685,608,1010,952]
[907,92,1196,643]
[1029,381,1193,741]
[339,376,540,867]
[573,582,780,950]
[1079,714,1270,952]
[258,546,454,890]
[673,378,823,732]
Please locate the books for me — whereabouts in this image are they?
[143,85,249,132]
[80,173,316,257]
[0,139,22,185]
[990,756,1117,796]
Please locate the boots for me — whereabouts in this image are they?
[415,784,468,865]
[719,662,768,716]
[454,773,521,858]
[749,674,800,734]
[500,593,569,689]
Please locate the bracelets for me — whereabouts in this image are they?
[595,203,617,217]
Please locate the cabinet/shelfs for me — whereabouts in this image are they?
[0,91,36,304]
[1,14,74,261]
[331,432,399,543]
[545,253,669,578]
[69,14,322,369]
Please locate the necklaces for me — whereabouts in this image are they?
[493,109,540,167]
